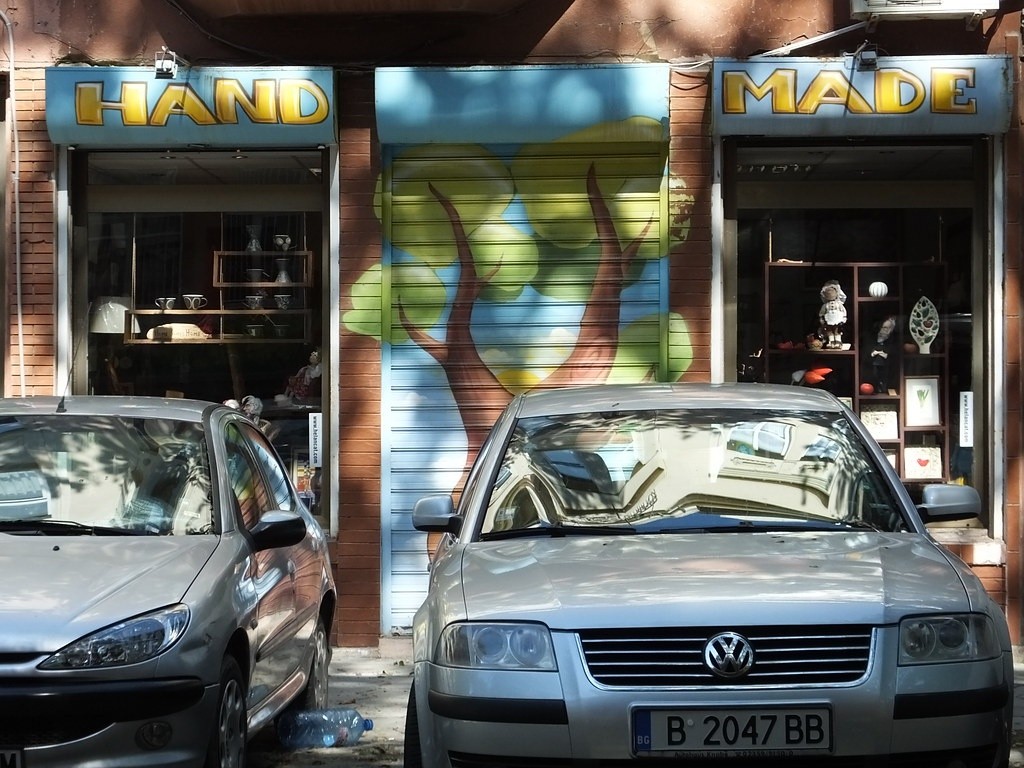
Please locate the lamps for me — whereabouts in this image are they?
[842,51,881,72]
[89,296,141,362]
[155,45,193,79]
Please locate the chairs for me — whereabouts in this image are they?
[514,449,614,530]
[128,473,215,531]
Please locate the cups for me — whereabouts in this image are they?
[241,296,264,310]
[274,325,290,337]
[243,325,265,337]
[242,269,262,282]
[273,295,295,309]
[155,298,176,310]
[273,235,291,251]
[183,295,207,310]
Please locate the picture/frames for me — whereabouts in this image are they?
[904,444,945,479]
[905,376,944,427]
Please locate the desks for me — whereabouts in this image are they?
[260,410,322,479]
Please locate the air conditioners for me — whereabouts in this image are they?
[848,0,999,22]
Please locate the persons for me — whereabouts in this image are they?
[864,313,895,395]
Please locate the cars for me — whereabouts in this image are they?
[403,381,1015,768]
[1,392,339,768]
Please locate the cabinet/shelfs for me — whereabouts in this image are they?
[124,212,314,346]
[762,259,951,486]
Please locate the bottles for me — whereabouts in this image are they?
[276,706,373,748]
